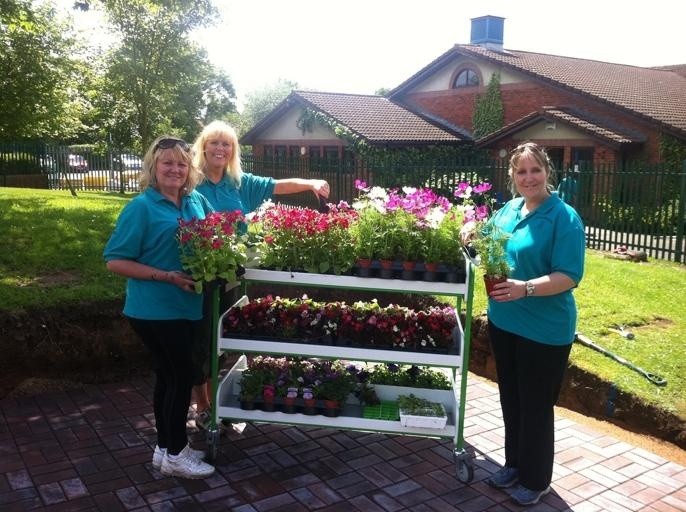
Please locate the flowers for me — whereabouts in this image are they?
[454,182,515,279]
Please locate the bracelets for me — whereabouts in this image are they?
[526,280,535,296]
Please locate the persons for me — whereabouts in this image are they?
[480,142,585,506]
[102,135,216,480]
[557,164,581,207]
[191,120,331,436]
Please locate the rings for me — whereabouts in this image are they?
[508,294,511,297]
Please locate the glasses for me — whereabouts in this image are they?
[509,145,546,155]
[152,139,190,155]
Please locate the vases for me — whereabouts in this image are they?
[240,399,339,411]
[483,274,507,296]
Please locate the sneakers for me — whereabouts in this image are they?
[489,466,520,488]
[510,484,551,505]
[152,444,205,470]
[160,445,215,480]
[196,408,227,437]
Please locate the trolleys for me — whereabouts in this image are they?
[206,252,475,482]
